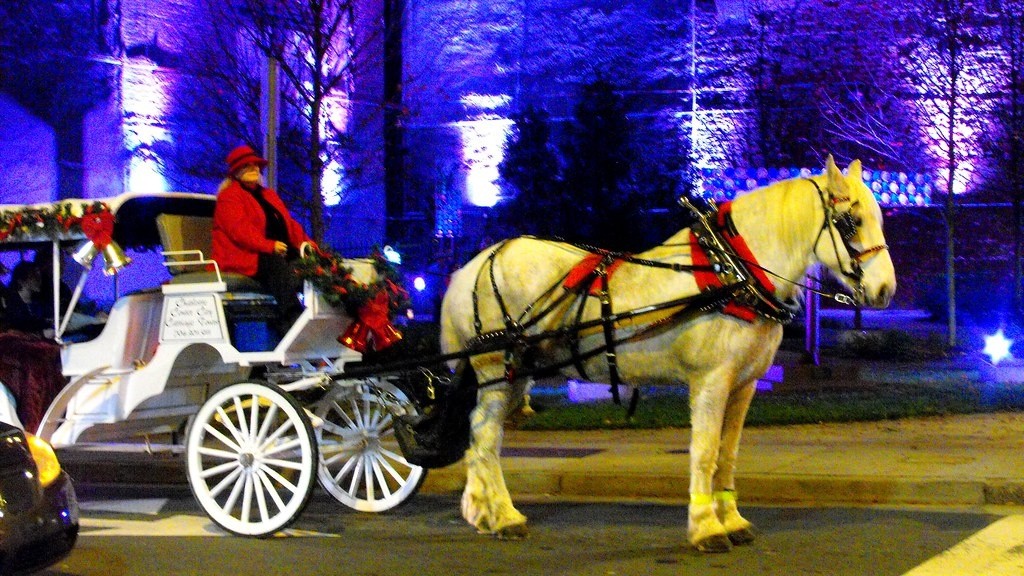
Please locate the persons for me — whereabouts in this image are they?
[204,145,319,343]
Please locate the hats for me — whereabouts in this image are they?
[226,145,270,174]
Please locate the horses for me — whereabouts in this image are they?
[439,151,898,553]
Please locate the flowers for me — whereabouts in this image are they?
[297,243,411,318]
[0,201,113,243]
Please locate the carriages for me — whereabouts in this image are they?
[0,152,898,555]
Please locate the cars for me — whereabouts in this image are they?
[0,380,79,576]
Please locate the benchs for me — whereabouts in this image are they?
[157,214,274,306]
